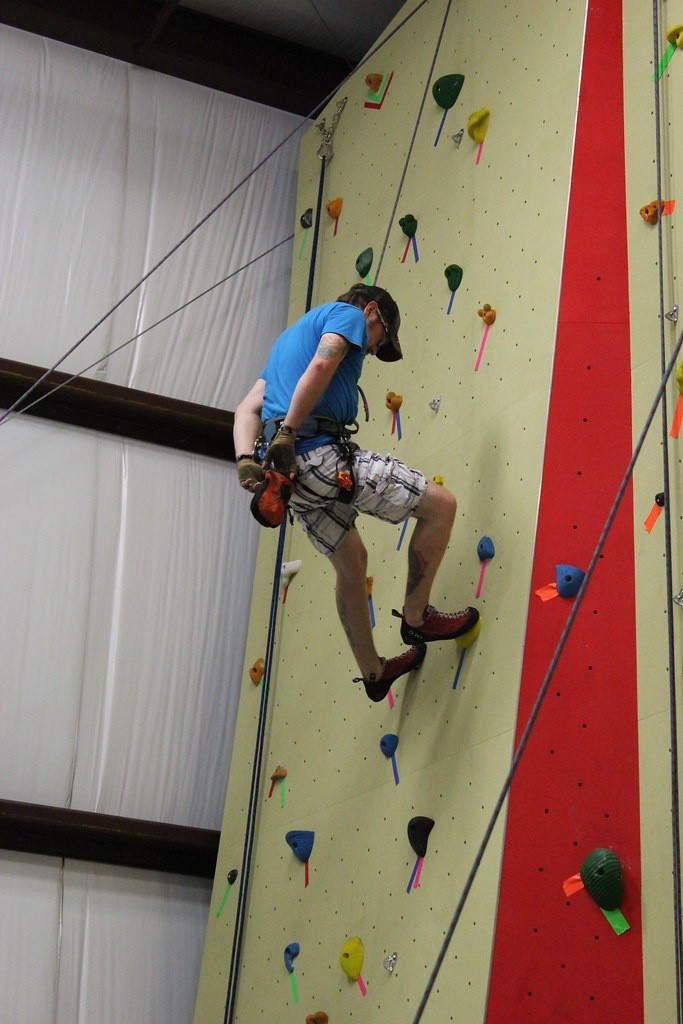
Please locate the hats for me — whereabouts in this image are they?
[356,285,403,363]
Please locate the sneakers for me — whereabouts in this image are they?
[352,643,426,702]
[391,605,479,645]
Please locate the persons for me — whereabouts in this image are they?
[232,282,481,701]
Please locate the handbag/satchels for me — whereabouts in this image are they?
[250,470,295,528]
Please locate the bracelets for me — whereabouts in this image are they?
[236,454,252,461]
